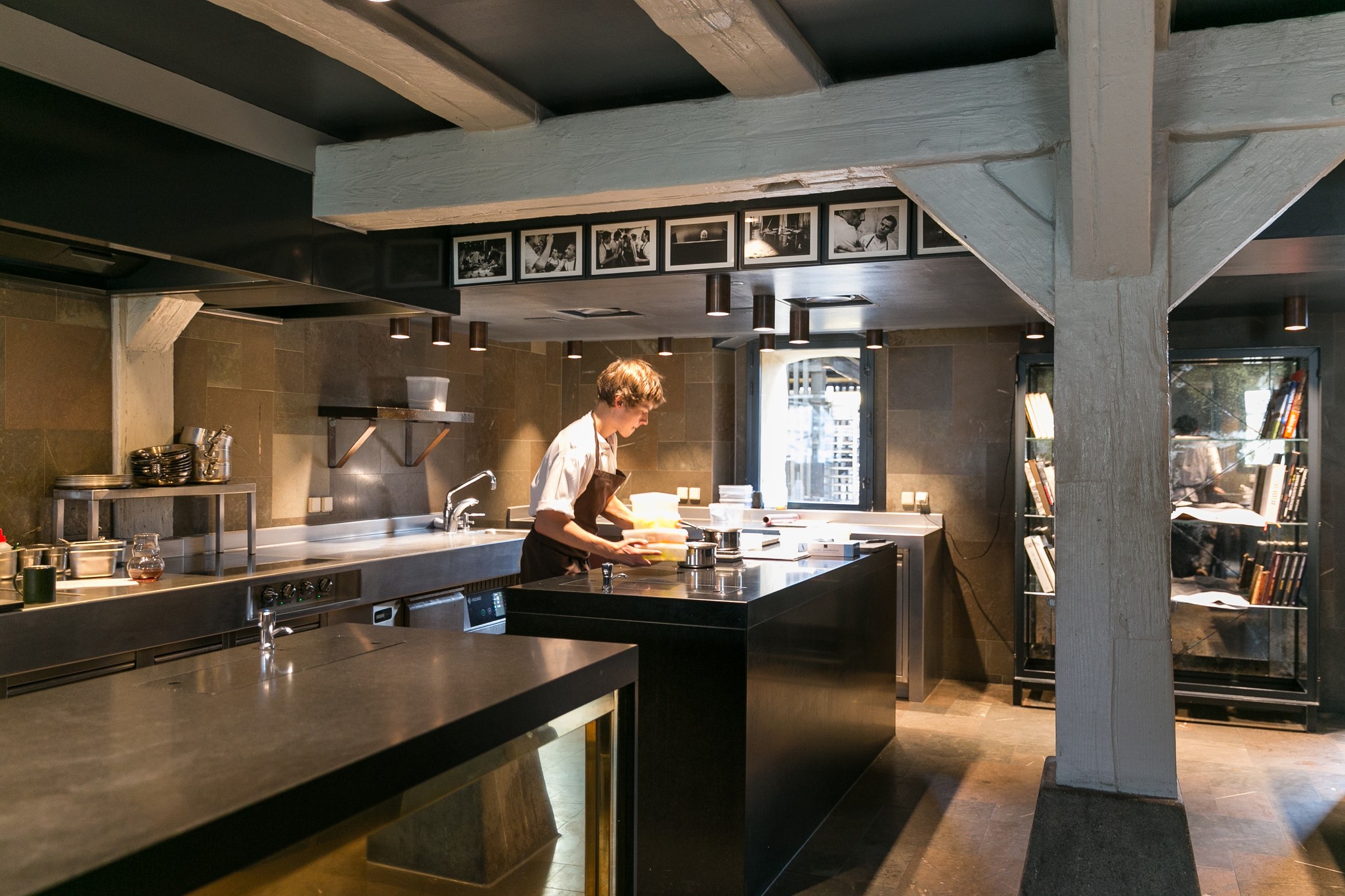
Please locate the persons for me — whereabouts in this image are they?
[834,208,898,253]
[598,230,650,269]
[519,358,667,584]
[458,244,507,278]
[1169,415,1227,577]
[697,230,712,242]
[525,233,576,274]
[783,234,804,249]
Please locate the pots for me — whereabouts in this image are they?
[129,444,192,487]
[677,542,718,568]
[680,520,743,550]
[176,425,234,484]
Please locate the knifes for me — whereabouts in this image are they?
[859,539,886,543]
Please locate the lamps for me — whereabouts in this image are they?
[788,311,810,345]
[468,322,488,351]
[759,334,775,352]
[752,296,775,332]
[866,329,883,349]
[657,336,673,357]
[432,316,452,345]
[705,275,731,316]
[389,317,411,339]
[1282,295,1308,331]
[1025,322,1045,341]
[567,340,583,358]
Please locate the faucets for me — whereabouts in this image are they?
[257,608,294,650]
[601,562,629,591]
[446,498,480,533]
[442,469,497,531]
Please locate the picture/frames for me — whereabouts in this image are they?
[911,201,973,260]
[587,215,661,280]
[662,211,738,276]
[824,196,912,264]
[449,229,515,289]
[517,221,587,285]
[741,203,822,270]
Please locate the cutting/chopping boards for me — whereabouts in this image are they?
[843,540,894,553]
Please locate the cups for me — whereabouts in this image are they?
[13,565,56,604]
[752,492,762,508]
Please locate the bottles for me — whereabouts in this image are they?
[776,482,788,510]
[126,533,165,582]
[0,528,11,552]
[11,541,21,550]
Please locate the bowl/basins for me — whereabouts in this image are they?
[621,492,688,562]
[709,503,744,526]
[718,484,753,509]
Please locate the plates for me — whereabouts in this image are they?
[54,474,134,490]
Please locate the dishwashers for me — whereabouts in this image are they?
[464,584,506,634]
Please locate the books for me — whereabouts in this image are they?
[1237,540,1308,590]
[1261,369,1306,439]
[1248,551,1308,606]
[1024,392,1055,438]
[1252,450,1309,523]
[1024,535,1055,593]
[1024,458,1056,515]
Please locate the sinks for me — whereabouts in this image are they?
[465,529,530,537]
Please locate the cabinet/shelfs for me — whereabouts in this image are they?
[1012,344,1324,732]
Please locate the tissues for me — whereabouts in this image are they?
[798,534,860,557]
